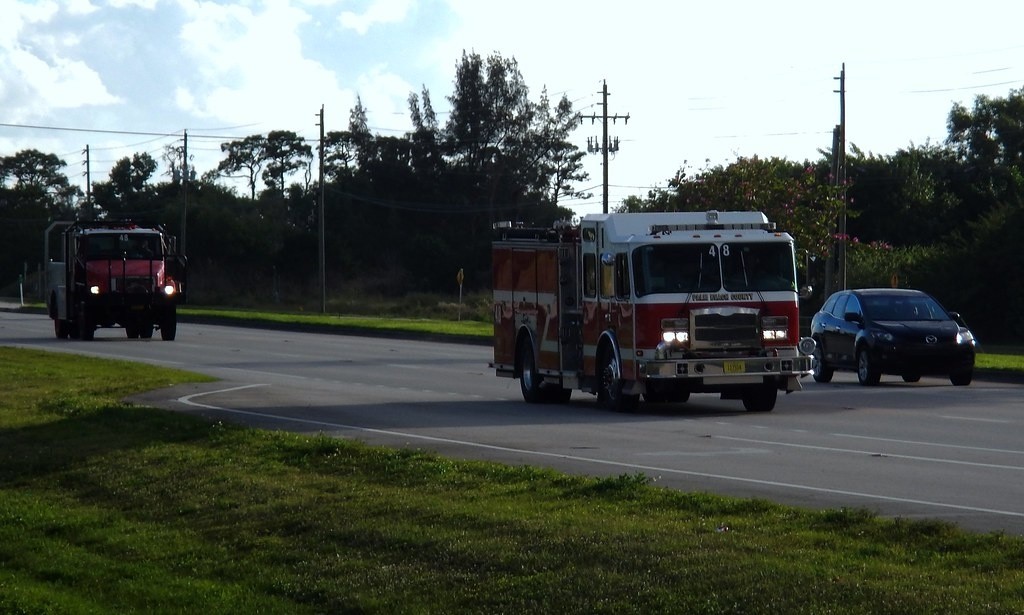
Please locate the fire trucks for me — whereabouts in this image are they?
[489,210,816,411]
[44,218,184,341]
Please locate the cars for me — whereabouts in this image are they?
[811,289,979,387]
